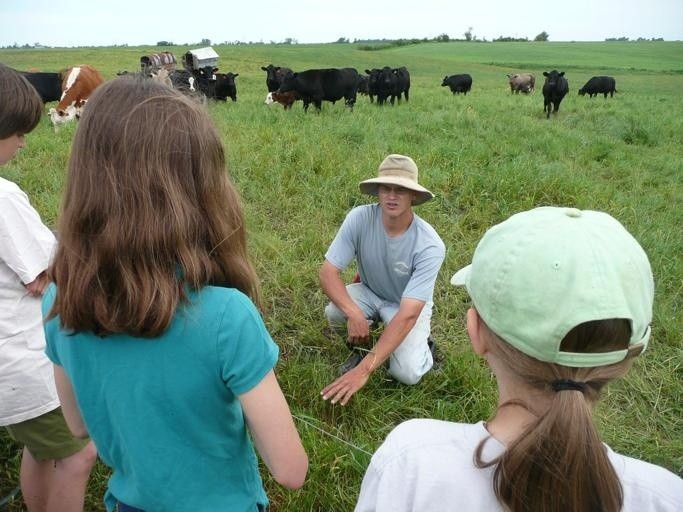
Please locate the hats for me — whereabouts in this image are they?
[451,206,655,368]
[360,154,435,205]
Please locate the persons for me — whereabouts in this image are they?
[355,206,682,511]
[315,153,448,405]
[42,75,309,510]
[0,66,98,510]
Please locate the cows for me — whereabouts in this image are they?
[116,65,239,103]
[48,63,105,134]
[261,64,411,114]
[25,70,63,105]
[542,70,568,119]
[578,75,617,98]
[506,73,536,96]
[441,74,472,96]
